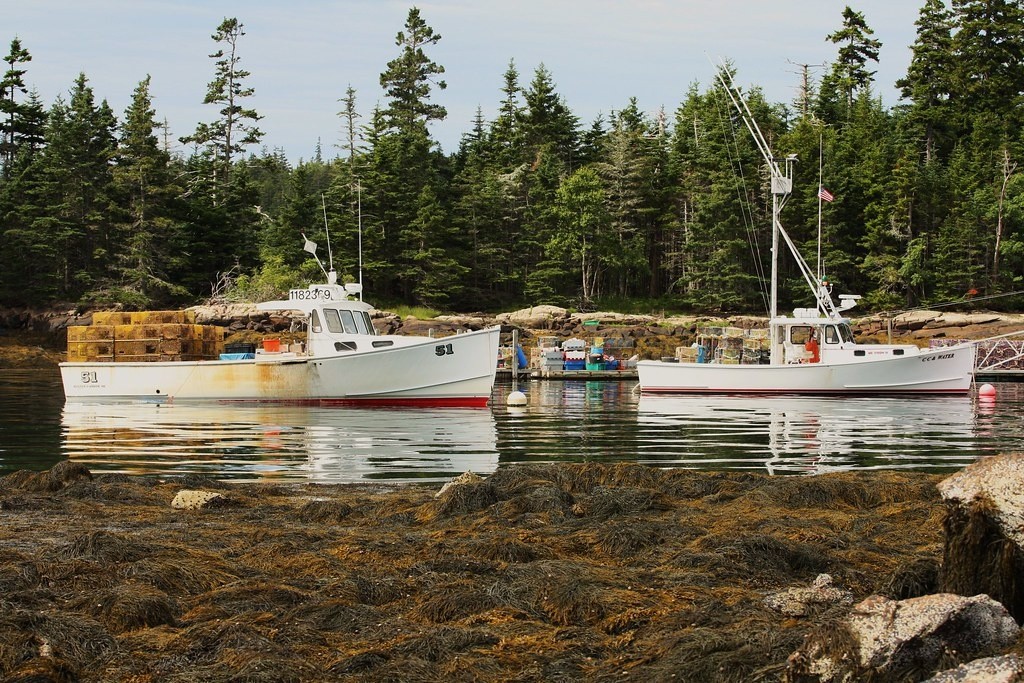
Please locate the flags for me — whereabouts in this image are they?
[818,188,833,202]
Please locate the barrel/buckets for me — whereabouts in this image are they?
[697,348,705,363]
[261,337,280,352]
[509,344,527,368]
[224,343,255,353]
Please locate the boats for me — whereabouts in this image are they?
[56,176,505,414]
[635,48,976,402]
